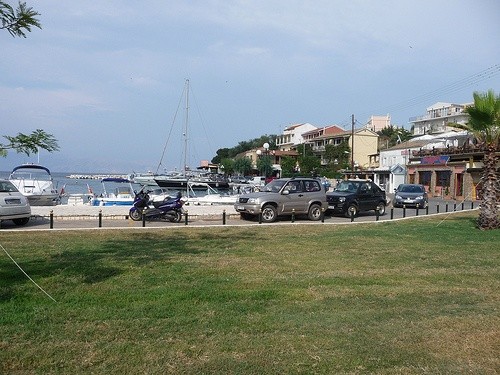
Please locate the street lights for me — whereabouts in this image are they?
[256,143,275,178]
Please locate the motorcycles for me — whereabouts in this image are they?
[129,189,185,223]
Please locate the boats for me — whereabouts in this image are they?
[91,79,256,205]
[8,148,60,206]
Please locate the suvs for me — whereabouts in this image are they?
[326,179,387,218]
[234,177,328,222]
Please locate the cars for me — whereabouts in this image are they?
[0,179,31,225]
[392,184,429,209]
[210,173,331,191]
